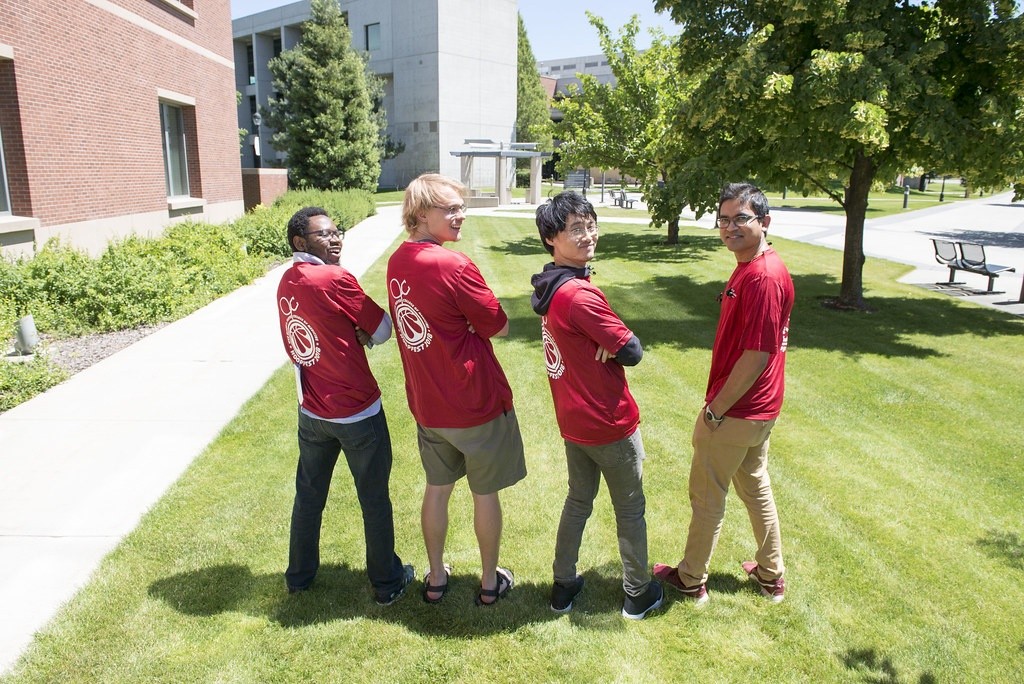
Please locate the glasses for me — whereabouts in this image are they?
[558,225,600,238]
[716,214,765,228]
[426,205,467,215]
[298,229,344,240]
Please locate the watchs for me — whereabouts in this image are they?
[706,404,724,423]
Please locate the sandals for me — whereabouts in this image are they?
[474,566,514,607]
[423,564,451,604]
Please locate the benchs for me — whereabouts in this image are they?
[620,188,638,208]
[608,189,620,206]
[929,237,1015,294]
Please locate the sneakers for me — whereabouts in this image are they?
[375,564,415,607]
[742,562,785,604]
[550,573,585,614]
[622,580,664,620]
[653,563,709,606]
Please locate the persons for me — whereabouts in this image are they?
[652,186,794,600]
[531,191,664,619]
[276,207,417,607]
[387,175,527,606]
